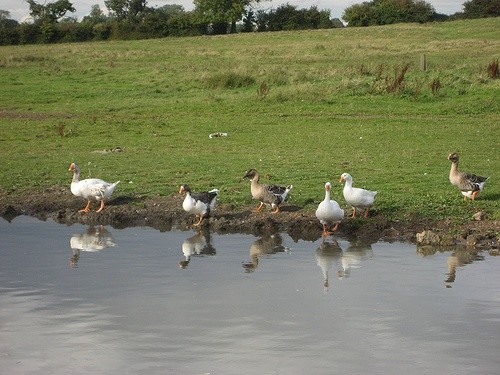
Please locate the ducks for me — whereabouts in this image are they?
[69,225,117,265]
[179,229,217,268]
[178,184,220,226]
[337,243,374,279]
[243,168,293,214]
[241,232,290,271]
[448,153,491,201]
[315,182,344,237]
[339,173,378,219]
[68,163,121,213]
[315,238,343,290]
